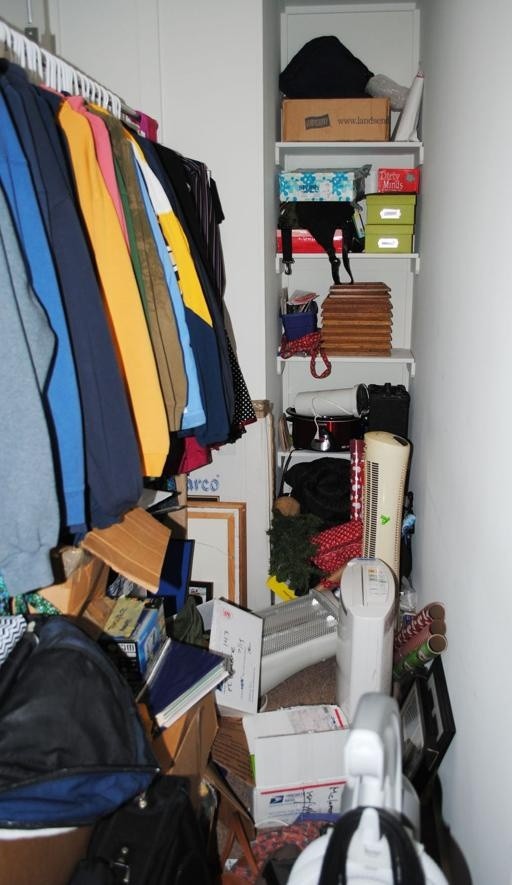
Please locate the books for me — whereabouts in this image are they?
[144,639,231,731]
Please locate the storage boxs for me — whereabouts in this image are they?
[280,98,391,142]
[276,167,420,254]
[0,690,218,885]
[13,504,172,643]
[242,704,355,828]
[171,597,264,720]
[105,593,167,676]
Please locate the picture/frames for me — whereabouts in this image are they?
[399,653,457,792]
[186,493,246,612]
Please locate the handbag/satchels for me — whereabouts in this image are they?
[296,202,355,285]
[279,330,332,379]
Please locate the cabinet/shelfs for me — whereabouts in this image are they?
[274,140,423,467]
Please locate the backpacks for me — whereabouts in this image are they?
[1,615,174,830]
[68,776,220,884]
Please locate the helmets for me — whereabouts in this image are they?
[286,805,449,885]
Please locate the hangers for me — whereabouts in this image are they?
[0,19,158,144]
